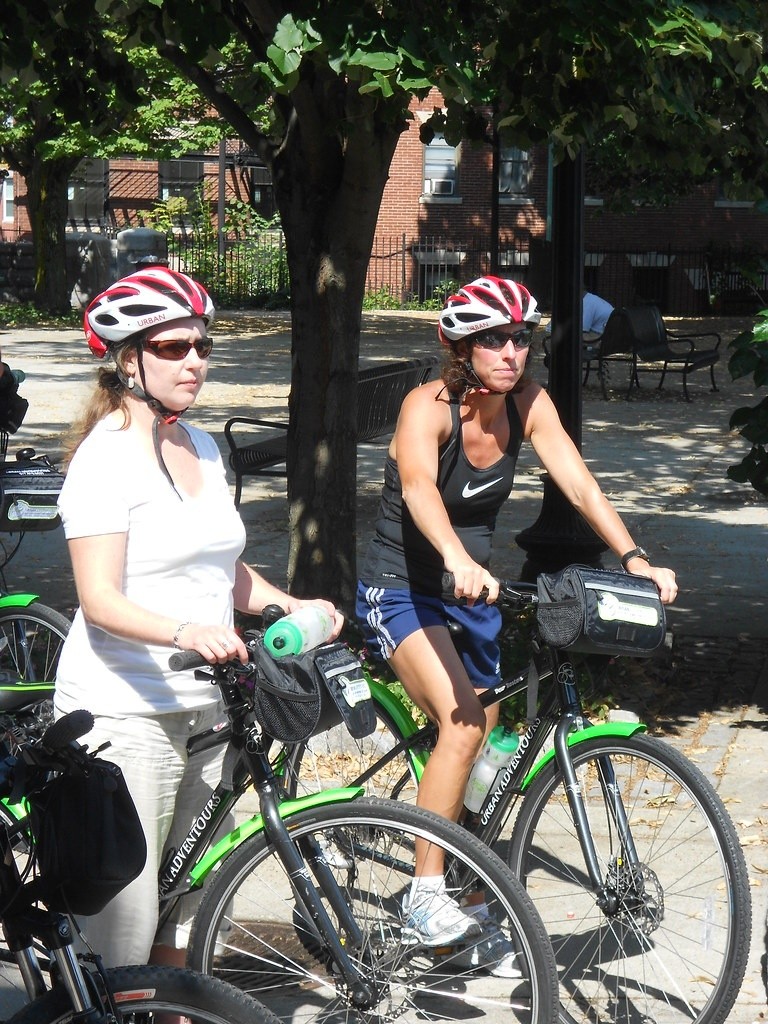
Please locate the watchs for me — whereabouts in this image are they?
[621,546,650,571]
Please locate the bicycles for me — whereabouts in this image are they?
[0,704,287,1023]
[282,564,754,1024]
[0,604,559,1024]
[0,361,75,742]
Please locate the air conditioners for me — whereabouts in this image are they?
[431,179,455,196]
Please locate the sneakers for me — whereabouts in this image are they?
[400,882,484,948]
[440,913,524,979]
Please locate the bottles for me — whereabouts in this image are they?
[263,604,333,658]
[462,724,519,814]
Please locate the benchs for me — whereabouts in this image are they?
[225,356,439,513]
[542,307,721,403]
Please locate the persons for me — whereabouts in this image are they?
[356,276,676,977]
[544,290,614,368]
[55,265,346,1024]
[0,350,16,409]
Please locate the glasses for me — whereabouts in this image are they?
[473,328,536,350]
[140,337,213,361]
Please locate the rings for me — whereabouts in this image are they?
[220,639,229,649]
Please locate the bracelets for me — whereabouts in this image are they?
[174,621,189,649]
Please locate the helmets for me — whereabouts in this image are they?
[437,276,541,350]
[83,266,216,359]
